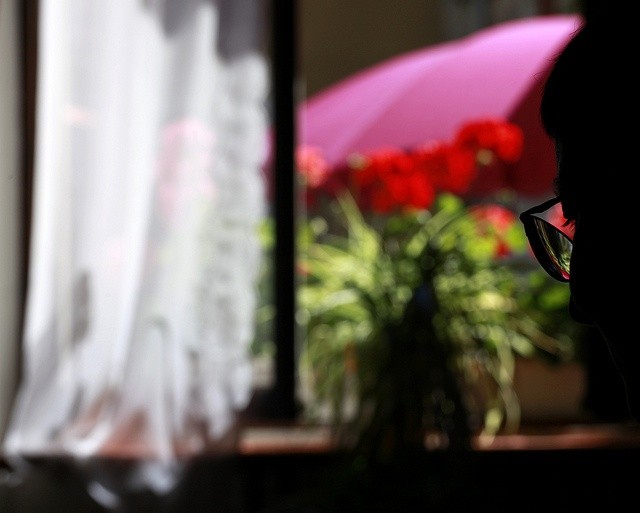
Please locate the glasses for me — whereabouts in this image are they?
[520,195,577,281]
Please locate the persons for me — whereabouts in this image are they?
[520,9,640,389]
